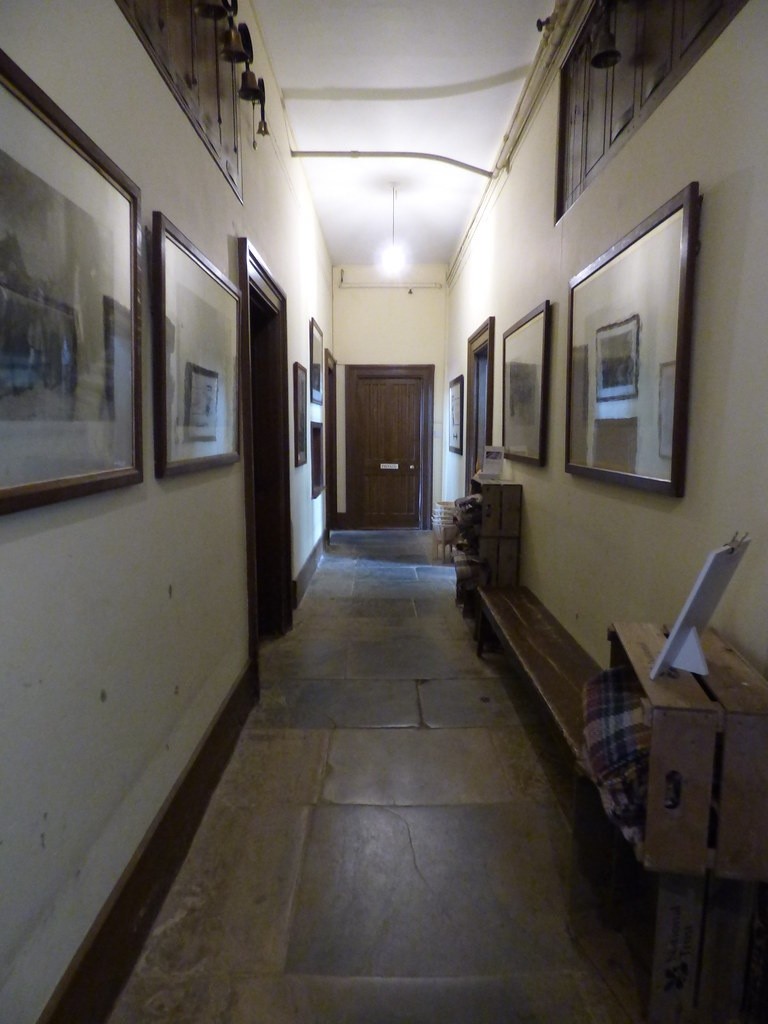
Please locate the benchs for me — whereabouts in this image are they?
[476,584,758,1024]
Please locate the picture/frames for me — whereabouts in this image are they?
[150,209,244,480]
[501,301,550,468]
[0,48,143,513]
[310,317,324,406]
[293,361,307,467]
[448,375,463,454]
[562,181,700,494]
[310,422,327,498]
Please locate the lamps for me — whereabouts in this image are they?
[202,0,226,19]
[591,1,620,69]
[256,78,269,136]
[239,63,261,101]
[382,180,406,275]
[223,17,246,63]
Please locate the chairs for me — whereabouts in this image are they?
[431,501,456,564]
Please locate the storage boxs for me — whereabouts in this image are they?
[608,620,767,877]
[470,477,522,584]
